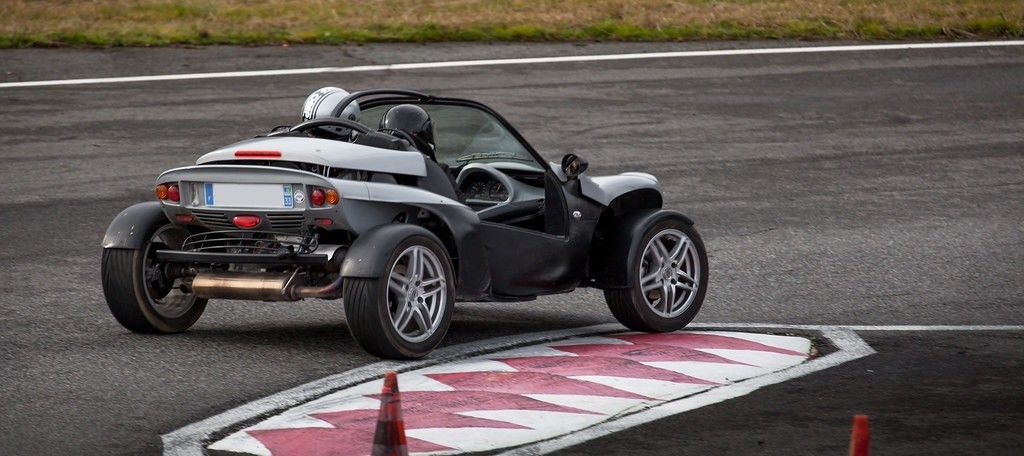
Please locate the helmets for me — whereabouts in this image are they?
[300,87,361,144]
[379,104,436,154]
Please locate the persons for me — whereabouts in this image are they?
[300,87,363,148]
[381,104,471,208]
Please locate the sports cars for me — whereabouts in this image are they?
[107,89,710,363]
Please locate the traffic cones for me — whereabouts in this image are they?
[370,370,411,456]
